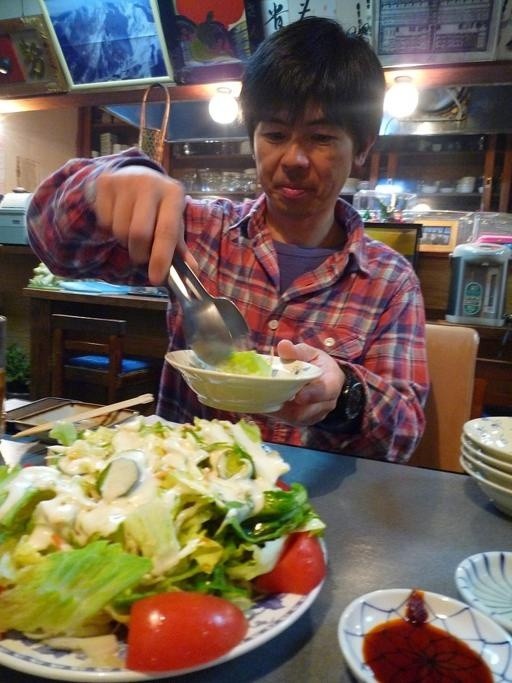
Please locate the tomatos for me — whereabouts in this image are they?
[254,532,326,596]
[125,592,246,671]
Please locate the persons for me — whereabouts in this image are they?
[25,16,430,464]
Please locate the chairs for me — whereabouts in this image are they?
[48,306,161,413]
[400,320,482,473]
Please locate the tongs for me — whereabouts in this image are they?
[164,251,252,364]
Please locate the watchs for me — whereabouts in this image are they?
[321,366,366,425]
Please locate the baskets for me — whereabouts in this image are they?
[139,82,171,163]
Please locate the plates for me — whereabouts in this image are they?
[453,548,512,630]
[455,175,476,193]
[457,413,512,515]
[0,514,327,681]
[336,587,511,683]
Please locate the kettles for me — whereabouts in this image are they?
[441,240,511,327]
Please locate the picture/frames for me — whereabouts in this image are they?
[155,0,262,86]
[36,0,182,96]
[0,11,72,99]
[367,0,502,71]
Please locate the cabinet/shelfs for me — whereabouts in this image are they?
[163,139,256,203]
[373,133,498,214]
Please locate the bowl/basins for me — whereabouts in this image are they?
[162,348,322,415]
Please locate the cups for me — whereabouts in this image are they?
[174,166,258,196]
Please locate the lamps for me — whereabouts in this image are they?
[374,174,406,213]
[380,73,419,121]
[205,84,239,125]
